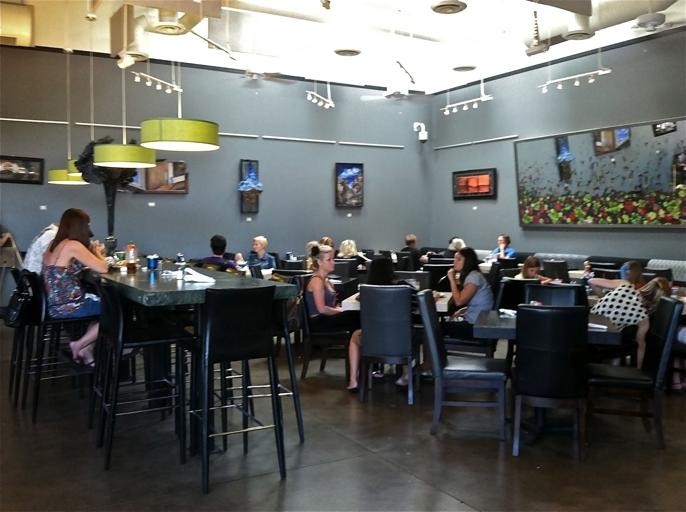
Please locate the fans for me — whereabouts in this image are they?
[221,47,297,89]
[621,0,686,43]
[360,73,433,103]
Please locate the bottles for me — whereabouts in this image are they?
[176,268,184,280]
[176,280,184,291]
[290,253,293,260]
[126,243,137,273]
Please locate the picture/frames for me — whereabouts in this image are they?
[238,159,264,214]
[453,169,497,200]
[592,127,631,157]
[513,116,686,231]
[652,121,677,137]
[0,155,44,185]
[130,160,189,194]
[335,163,364,208]
[555,135,574,181]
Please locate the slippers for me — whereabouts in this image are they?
[57,350,81,370]
[76,359,95,371]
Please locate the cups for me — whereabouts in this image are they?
[286,252,293,260]
[176,254,184,262]
[292,257,297,261]
[148,270,159,289]
[115,252,125,259]
[104,235,118,257]
[146,255,159,269]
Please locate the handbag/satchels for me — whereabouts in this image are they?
[5,293,38,328]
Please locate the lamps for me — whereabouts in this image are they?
[537,30,612,94]
[305,71,335,111]
[48,13,213,185]
[131,44,184,94]
[439,66,491,116]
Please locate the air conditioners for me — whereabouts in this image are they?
[0,3,36,49]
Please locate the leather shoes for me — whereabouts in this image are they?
[371,370,385,378]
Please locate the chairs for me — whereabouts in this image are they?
[4,268,304,494]
[223,247,684,464]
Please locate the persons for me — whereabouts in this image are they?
[197,234,243,274]
[0,221,15,248]
[41,207,102,371]
[418,246,496,377]
[443,238,467,258]
[400,232,431,271]
[344,254,411,392]
[235,235,277,280]
[333,239,372,272]
[514,254,686,391]
[303,240,386,380]
[21,218,62,293]
[318,237,335,248]
[481,233,518,264]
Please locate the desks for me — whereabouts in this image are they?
[85,258,303,464]
[472,309,623,445]
[341,291,453,388]
